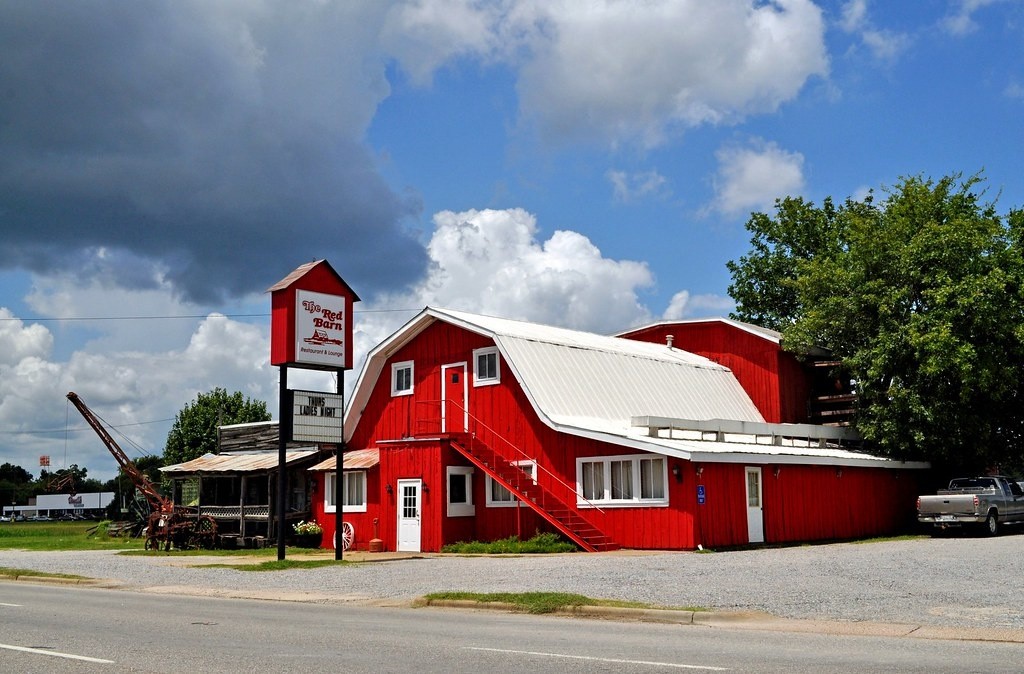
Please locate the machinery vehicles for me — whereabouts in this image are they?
[64,392,222,552]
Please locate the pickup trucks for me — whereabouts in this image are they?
[915,476,1024,536]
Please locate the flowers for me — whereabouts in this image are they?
[292,519,324,534]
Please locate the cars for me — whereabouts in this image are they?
[1,512,97,522]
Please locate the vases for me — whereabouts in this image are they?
[295,533,322,548]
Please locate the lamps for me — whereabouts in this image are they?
[421,482,430,493]
[672,463,683,483]
[385,482,393,494]
